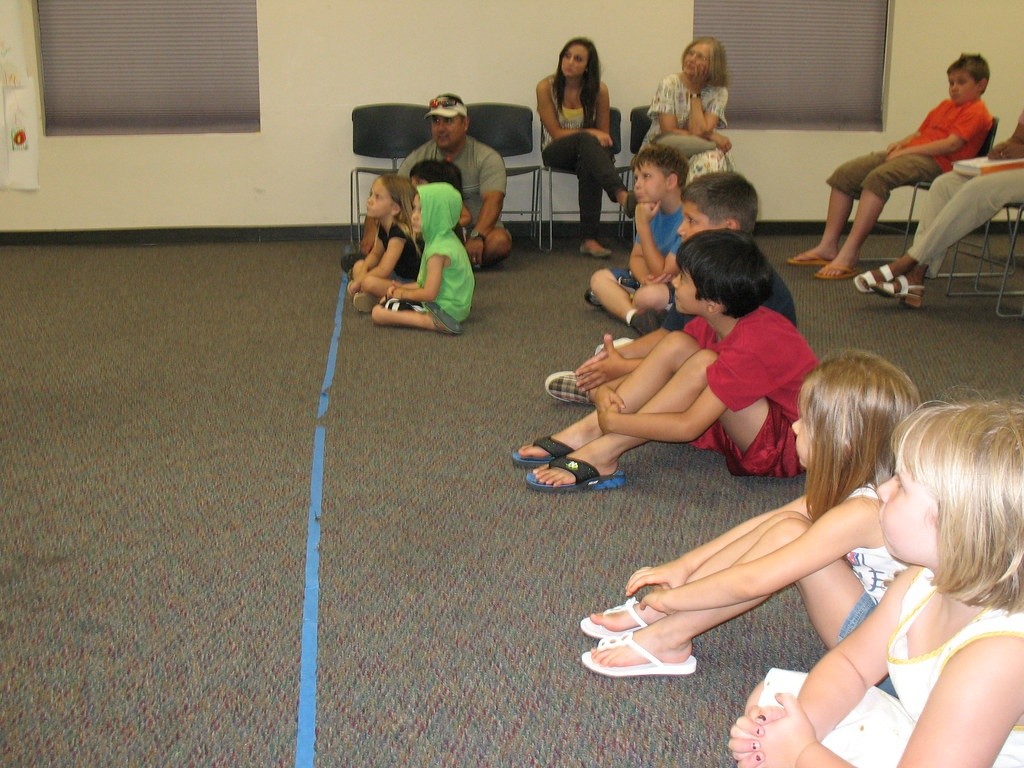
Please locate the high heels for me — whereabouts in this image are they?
[871,274,925,308]
[853,264,895,293]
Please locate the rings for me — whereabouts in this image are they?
[473,258,476,260]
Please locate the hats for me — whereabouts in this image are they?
[424,97,468,118]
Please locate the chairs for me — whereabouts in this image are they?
[852,116,1024,321]
[349,102,651,245]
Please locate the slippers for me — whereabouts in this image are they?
[580,596,648,637]
[815,264,860,280]
[425,302,464,335]
[787,252,832,265]
[581,632,697,676]
[512,435,575,467]
[525,456,625,493]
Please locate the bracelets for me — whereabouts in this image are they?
[690,93,701,98]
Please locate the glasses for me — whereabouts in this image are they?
[430,99,457,108]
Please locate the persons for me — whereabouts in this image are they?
[584,144,689,333]
[340,93,513,273]
[537,37,638,257]
[728,391,1024,768]
[511,225,821,492]
[347,160,471,312]
[580,349,923,699]
[362,182,474,335]
[545,172,798,407]
[853,111,1024,307]
[639,36,733,182]
[786,54,993,279]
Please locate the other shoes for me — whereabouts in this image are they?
[625,190,638,219]
[628,307,667,335]
[584,287,603,306]
[579,246,614,258]
[353,292,377,313]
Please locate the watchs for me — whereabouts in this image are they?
[471,231,485,242]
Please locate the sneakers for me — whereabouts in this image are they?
[544,371,596,406]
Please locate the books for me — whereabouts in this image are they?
[952,156,1024,176]
